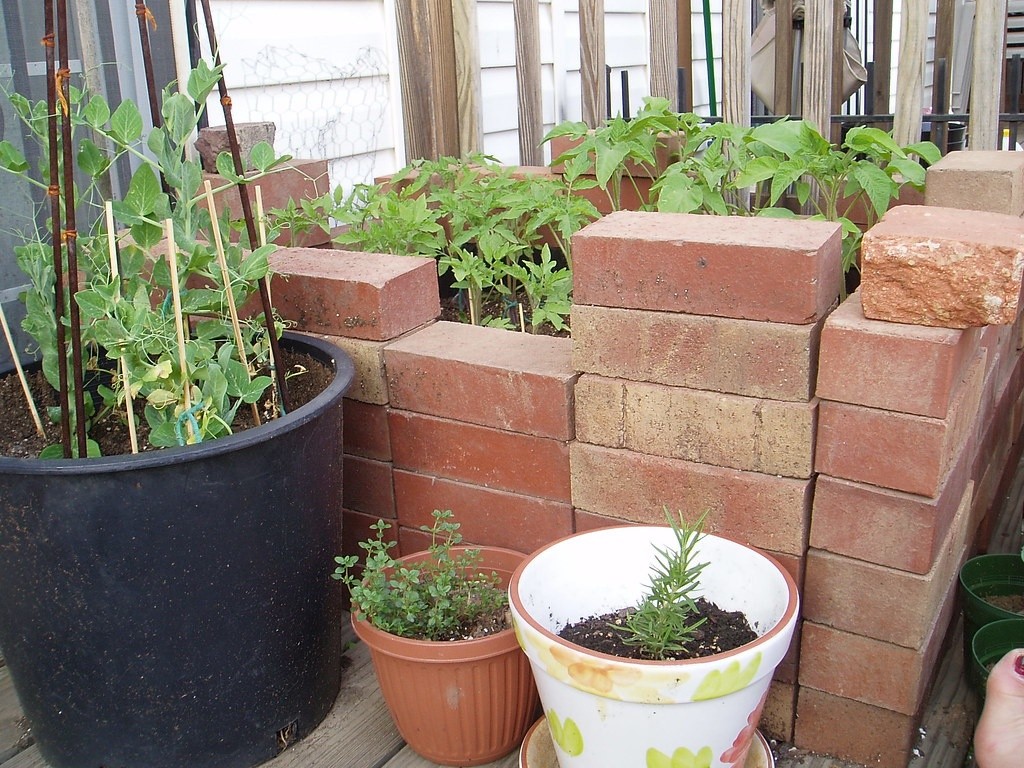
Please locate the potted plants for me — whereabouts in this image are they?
[507,503,802,768]
[0,32,356,768]
[332,508,542,768]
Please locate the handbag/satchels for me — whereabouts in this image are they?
[749,8,866,117]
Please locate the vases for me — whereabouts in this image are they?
[958,552,1024,676]
[972,618,1024,724]
